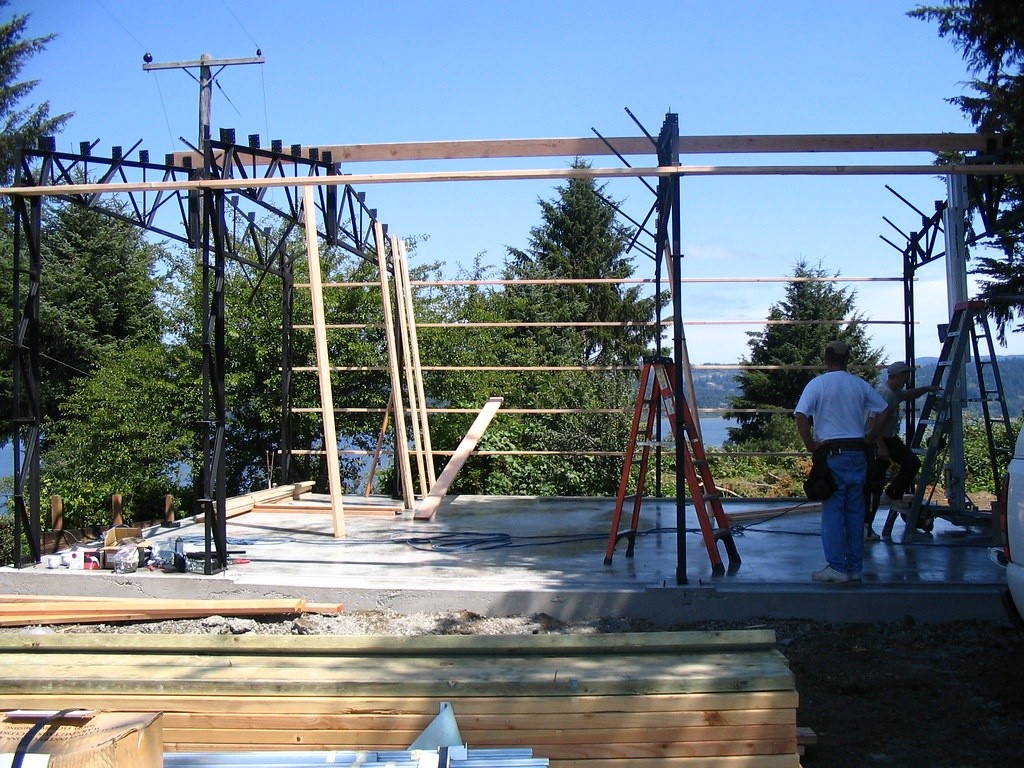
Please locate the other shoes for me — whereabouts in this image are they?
[863,524,880,540]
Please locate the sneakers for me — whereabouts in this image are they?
[812,565,848,582]
[849,574,860,579]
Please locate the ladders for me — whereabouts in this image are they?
[600,355,743,578]
[880,298,1018,546]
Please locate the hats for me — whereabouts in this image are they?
[888,362,915,375]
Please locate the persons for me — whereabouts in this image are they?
[866,362,940,542]
[792,339,893,582]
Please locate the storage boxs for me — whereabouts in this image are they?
[70,542,106,570]
[100,527,157,568]
[188,559,229,575]
[0,711,165,768]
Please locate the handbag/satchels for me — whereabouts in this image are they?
[803,450,835,500]
